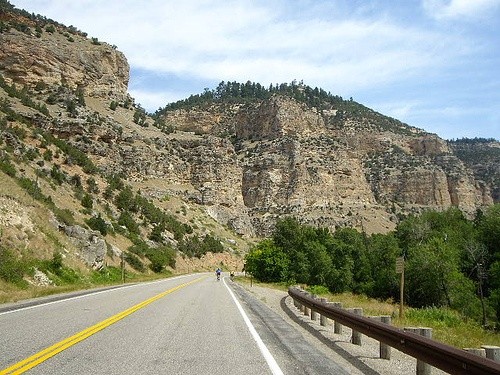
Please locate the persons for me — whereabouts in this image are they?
[215,268,221,279]
